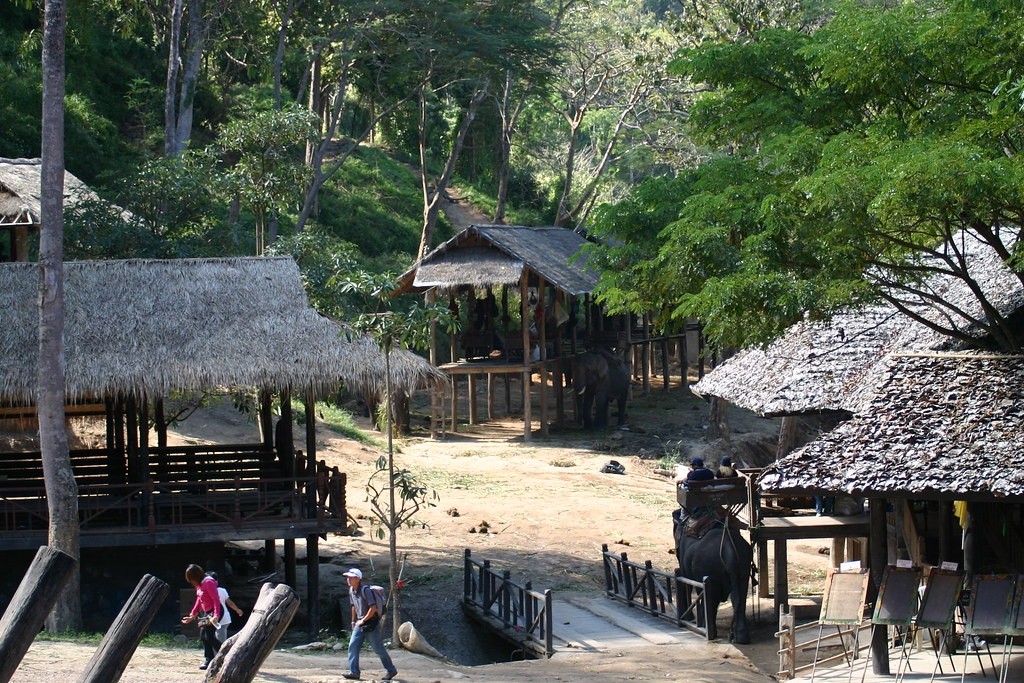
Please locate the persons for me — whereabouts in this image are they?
[341,568,398,680]
[206,570,243,644]
[685,457,715,480]
[715,455,738,479]
[181,564,225,670]
[814,494,835,518]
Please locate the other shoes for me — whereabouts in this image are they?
[199,663,208,670]
[341,671,360,679]
[382,670,397,679]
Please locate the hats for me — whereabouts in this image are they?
[342,568,362,579]
[721,456,731,466]
[690,457,704,466]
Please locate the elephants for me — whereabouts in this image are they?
[672,505,755,646]
[565,348,633,434]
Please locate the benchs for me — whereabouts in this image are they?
[461,334,553,363]
[583,331,626,356]
[675,477,748,510]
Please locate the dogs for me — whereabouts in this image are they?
[614,538,631,546]
[446,506,460,518]
[469,519,491,536]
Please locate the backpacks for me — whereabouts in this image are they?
[361,585,387,620]
